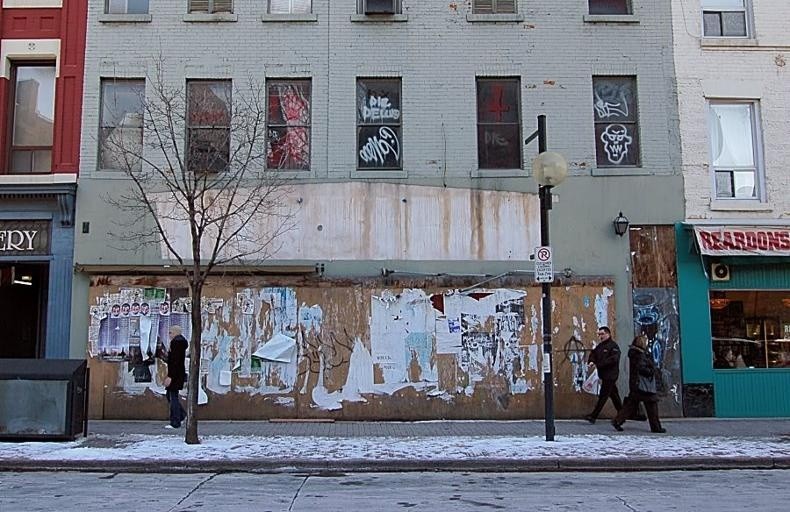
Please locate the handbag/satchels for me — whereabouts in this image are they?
[635,365,657,396]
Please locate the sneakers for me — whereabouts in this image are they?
[611,419,626,432]
[650,428,667,433]
[179,414,188,426]
[584,415,597,424]
[164,424,180,430]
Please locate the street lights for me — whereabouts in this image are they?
[526,115,567,441]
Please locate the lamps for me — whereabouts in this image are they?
[614,212,629,236]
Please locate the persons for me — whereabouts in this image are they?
[611,335,666,433]
[161,325,188,429]
[583,327,626,425]
[713,347,736,369]
[772,349,790,368]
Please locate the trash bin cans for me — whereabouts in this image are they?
[0,358,90,442]
